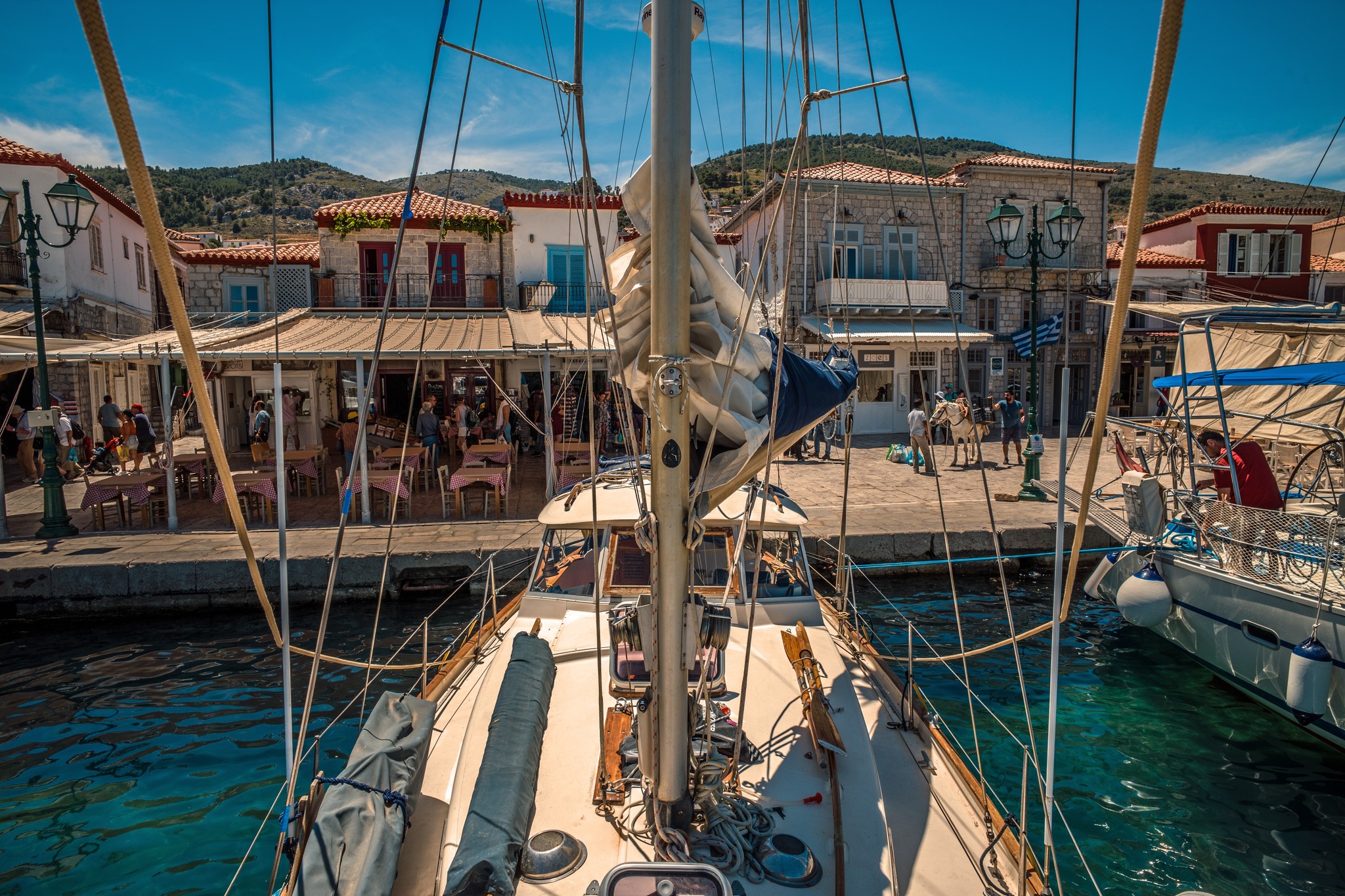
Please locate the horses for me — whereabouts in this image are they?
[929,400,983,467]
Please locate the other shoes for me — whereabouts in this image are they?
[926,469,938,473]
[783,445,830,459]
[84,464,90,469]
[530,451,541,456]
[1002,459,1009,464]
[1017,458,1024,464]
[914,471,919,473]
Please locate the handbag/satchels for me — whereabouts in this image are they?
[335,424,344,452]
[250,437,271,462]
[117,445,130,461]
[435,415,445,445]
[67,446,79,463]
[498,434,507,444]
[520,374,530,399]
[614,420,628,444]
[886,444,924,466]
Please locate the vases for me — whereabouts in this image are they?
[995,255,1007,265]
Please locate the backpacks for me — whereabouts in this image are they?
[59,415,85,440]
[32,426,44,450]
[509,405,522,435]
[465,405,480,432]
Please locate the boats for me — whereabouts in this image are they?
[1028,301,1345,767]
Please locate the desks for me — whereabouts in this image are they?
[373,448,432,493]
[554,442,598,464]
[152,453,208,500]
[449,468,511,520]
[212,472,292,525]
[558,465,599,490]
[1135,423,1169,457]
[462,445,518,468]
[448,423,483,457]
[1217,433,1253,442]
[339,470,409,522]
[80,474,167,531]
[262,450,333,498]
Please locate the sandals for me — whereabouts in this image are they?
[126,467,136,472]
[599,449,607,454]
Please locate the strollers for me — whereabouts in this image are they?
[86,436,125,476]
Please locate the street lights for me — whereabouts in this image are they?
[984,192,1086,504]
[0,172,101,540]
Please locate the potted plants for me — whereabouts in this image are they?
[327,269,336,278]
[483,275,497,307]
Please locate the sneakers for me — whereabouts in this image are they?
[20,469,86,484]
[115,470,141,476]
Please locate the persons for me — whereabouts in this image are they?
[415,395,444,481]
[531,384,564,456]
[1194,430,1286,551]
[956,390,967,401]
[479,393,523,462]
[920,392,932,431]
[336,412,361,476]
[271,386,302,451]
[783,443,796,457]
[593,391,611,454]
[808,413,833,458]
[928,391,949,444]
[243,390,270,473]
[630,393,643,443]
[987,390,1025,464]
[802,436,814,451]
[5,406,84,484]
[944,385,957,440]
[907,399,938,474]
[445,396,471,453]
[97,396,156,475]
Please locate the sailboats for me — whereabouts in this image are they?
[216,0,1066,892]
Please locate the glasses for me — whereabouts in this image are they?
[946,387,951,389]
[431,399,437,402]
[957,393,963,395]
[935,395,938,397]
[1202,443,1207,455]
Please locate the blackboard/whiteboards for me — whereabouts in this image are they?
[424,381,445,424]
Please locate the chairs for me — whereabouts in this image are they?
[1260,440,1345,494]
[1105,421,1214,470]
[84,417,607,533]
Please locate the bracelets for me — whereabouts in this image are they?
[450,417,452,420]
[560,385,577,436]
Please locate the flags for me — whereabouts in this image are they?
[1012,311,1064,359]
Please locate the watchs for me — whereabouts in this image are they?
[12,428,16,432]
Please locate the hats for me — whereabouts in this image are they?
[9,405,63,415]
[496,393,505,399]
[347,412,359,419]
[444,418,452,428]
[920,384,964,402]
[103,395,145,417]
